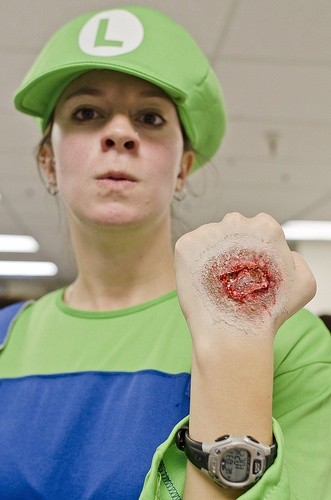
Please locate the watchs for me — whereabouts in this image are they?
[175,425,278,492]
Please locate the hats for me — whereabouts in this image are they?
[12,5,227,177]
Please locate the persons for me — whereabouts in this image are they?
[0,7,329,500]
